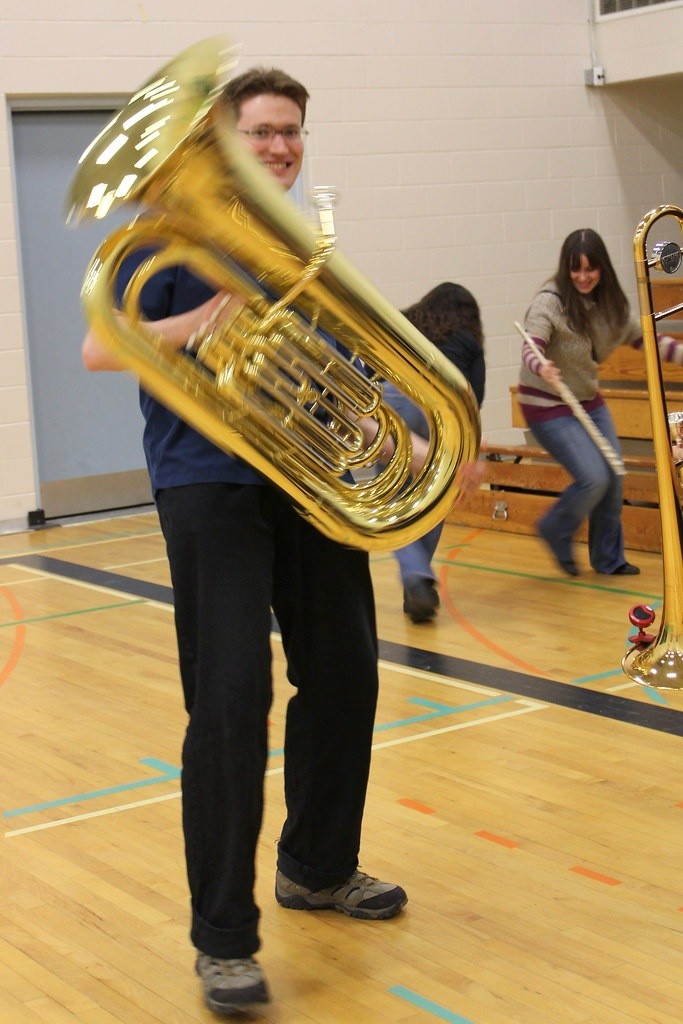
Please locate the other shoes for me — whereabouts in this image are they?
[616,562,640,575]
[403,579,437,623]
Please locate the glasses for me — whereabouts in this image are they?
[235,123,308,142]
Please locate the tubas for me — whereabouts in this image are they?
[61,33,483,554]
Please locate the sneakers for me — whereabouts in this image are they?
[274,864,408,919]
[195,951,270,1016]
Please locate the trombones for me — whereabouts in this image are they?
[616,202,683,692]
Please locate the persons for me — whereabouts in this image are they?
[518,229,683,576]
[365,282,486,625]
[80,68,409,1010]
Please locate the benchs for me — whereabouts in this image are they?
[445,278,683,555]
